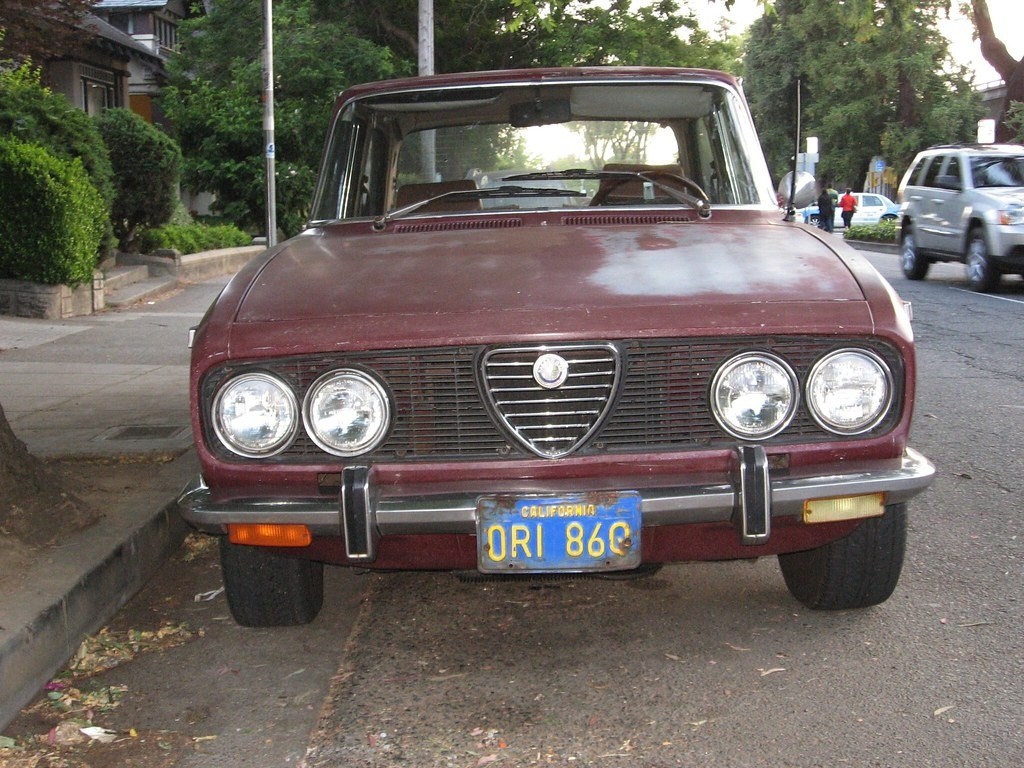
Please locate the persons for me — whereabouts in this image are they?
[839,188,858,229]
[826,183,838,220]
[817,190,833,232]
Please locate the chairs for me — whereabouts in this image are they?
[599,163,687,204]
[396,180,482,215]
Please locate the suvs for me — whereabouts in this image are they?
[898,143,1023,292]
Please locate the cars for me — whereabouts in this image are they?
[795,191,899,233]
[175,63,938,627]
